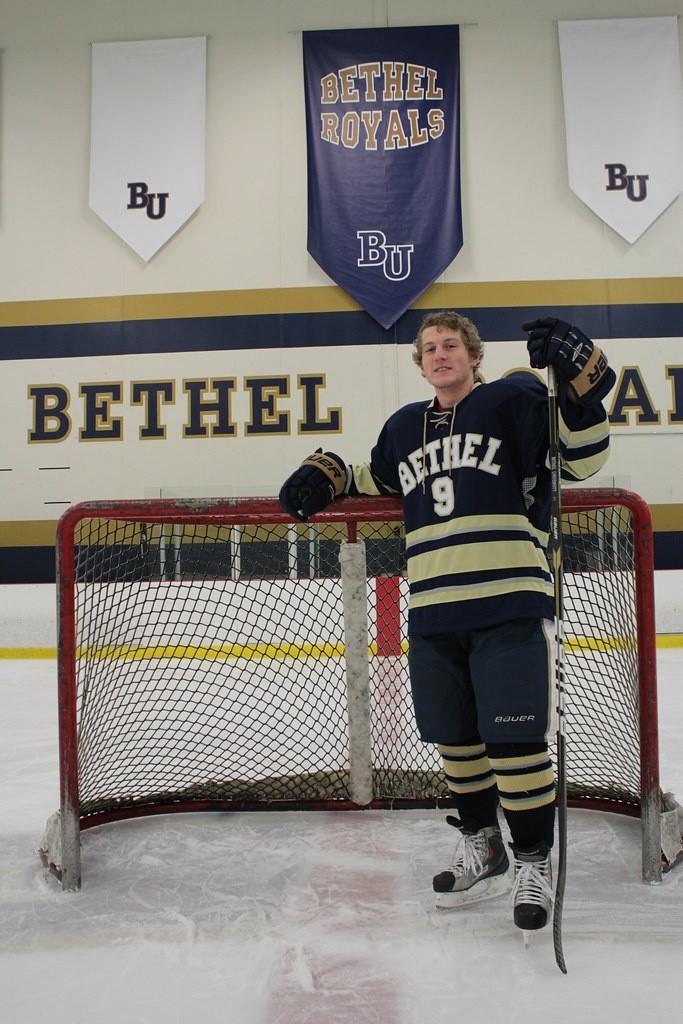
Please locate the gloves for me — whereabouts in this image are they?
[278,448,345,521]
[521,318,616,410]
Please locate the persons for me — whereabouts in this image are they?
[279,310,617,949]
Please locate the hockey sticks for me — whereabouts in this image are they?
[547,362,568,975]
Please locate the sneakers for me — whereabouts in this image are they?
[431,814,514,910]
[507,842,556,950]
